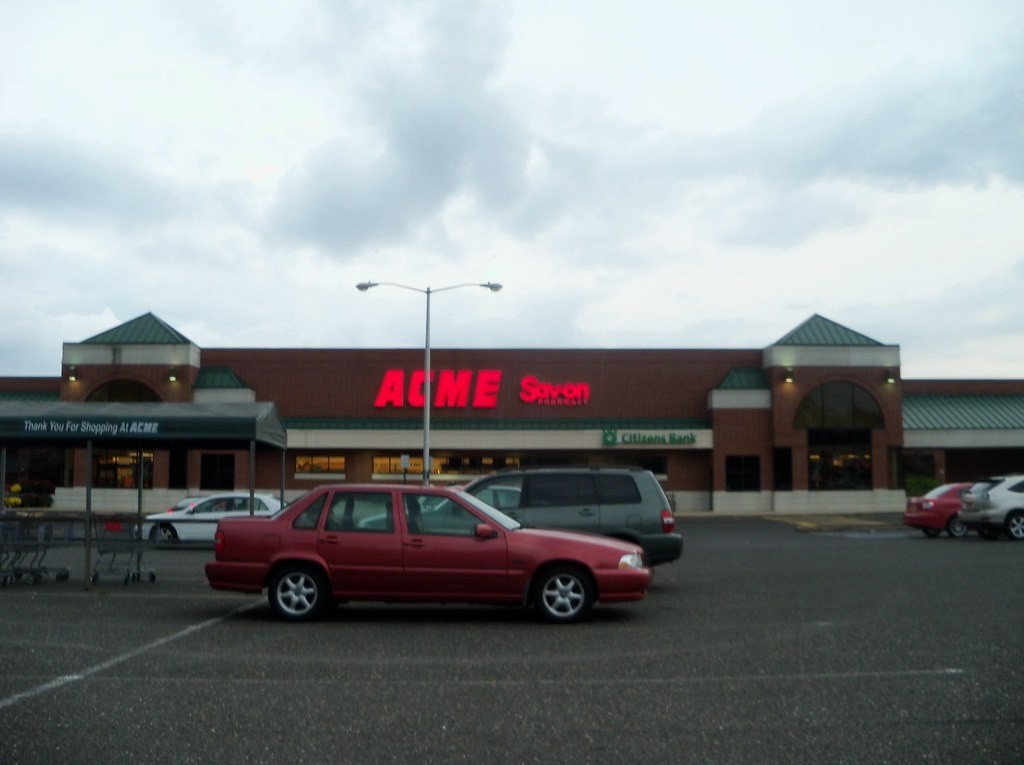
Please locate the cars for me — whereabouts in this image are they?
[903,483,975,538]
[134,493,306,550]
[205,483,655,622]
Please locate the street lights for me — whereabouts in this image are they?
[356,281,503,487]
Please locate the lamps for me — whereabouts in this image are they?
[69,370,76,382]
[785,370,793,383]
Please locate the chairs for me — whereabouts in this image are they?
[385,501,394,531]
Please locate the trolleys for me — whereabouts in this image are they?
[1,510,155,587]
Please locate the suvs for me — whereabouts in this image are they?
[357,463,683,567]
[957,475,1024,540]
